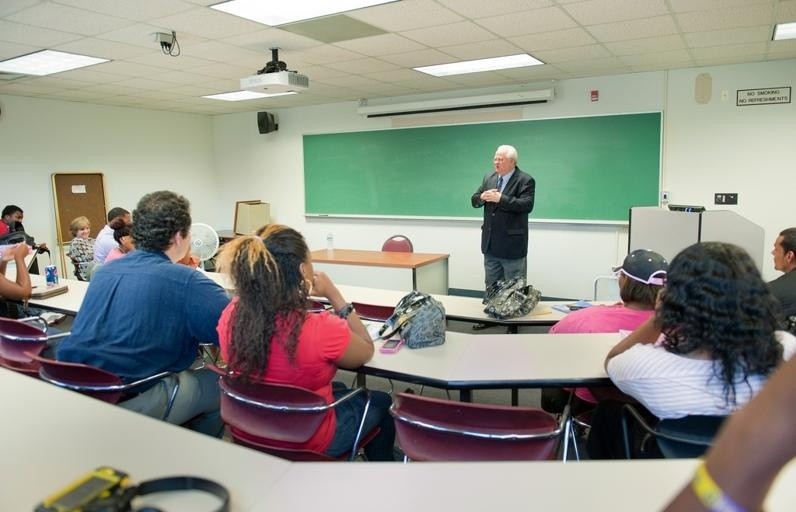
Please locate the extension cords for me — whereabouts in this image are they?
[152,32,174,47]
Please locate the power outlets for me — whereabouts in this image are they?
[715,193,737,204]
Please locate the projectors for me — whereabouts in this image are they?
[240,71,309,95]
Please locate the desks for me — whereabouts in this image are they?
[0,241,94,315]
[306,248,450,295]
[306,282,630,326]
[628,206,766,285]
[198,268,241,298]
[353,315,665,406]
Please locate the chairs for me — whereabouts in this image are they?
[391,389,586,462]
[0,315,72,374]
[188,221,219,272]
[624,401,736,458]
[24,349,181,422]
[205,362,384,461]
[383,235,411,254]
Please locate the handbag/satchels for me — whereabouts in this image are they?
[377,290,446,349]
[482,273,542,320]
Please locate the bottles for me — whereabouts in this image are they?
[45,265,58,289]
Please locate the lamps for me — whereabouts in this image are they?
[201,91,295,103]
[1,48,112,80]
[411,53,546,78]
[772,20,791,43]
[208,1,402,28]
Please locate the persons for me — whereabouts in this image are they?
[661,349,796,512]
[104,219,134,265]
[216,224,397,463]
[471,144,536,295]
[0,242,33,300]
[765,227,795,336]
[0,204,46,275]
[550,248,668,415]
[604,240,796,421]
[68,215,94,281]
[55,190,233,439]
[93,208,130,265]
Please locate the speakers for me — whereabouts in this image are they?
[256,110,275,135]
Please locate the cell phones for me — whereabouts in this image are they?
[380,338,403,354]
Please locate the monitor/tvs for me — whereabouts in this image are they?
[668,204,705,212]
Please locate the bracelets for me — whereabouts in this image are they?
[334,303,354,319]
[686,456,741,512]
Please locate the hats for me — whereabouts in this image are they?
[612,249,669,286]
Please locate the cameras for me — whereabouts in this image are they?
[34,466,131,512]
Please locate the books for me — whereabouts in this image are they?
[551,300,593,316]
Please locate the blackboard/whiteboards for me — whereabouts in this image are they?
[302,112,663,226]
